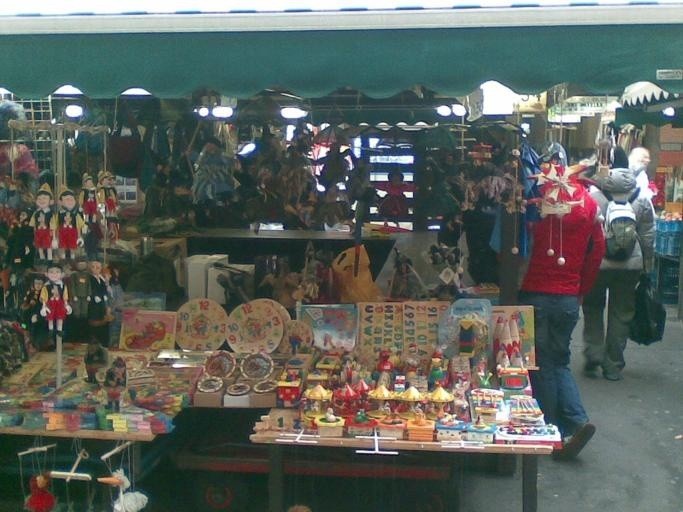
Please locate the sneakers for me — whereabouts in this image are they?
[586,352,620,382]
[552,422,595,461]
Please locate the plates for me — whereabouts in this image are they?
[241,352,274,379]
[254,298,292,320]
[175,299,227,351]
[254,380,277,393]
[202,349,235,378]
[196,376,224,392]
[225,303,284,354]
[226,383,251,395]
[277,320,313,354]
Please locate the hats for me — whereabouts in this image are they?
[609,145,628,169]
[545,141,568,166]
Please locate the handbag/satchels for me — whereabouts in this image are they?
[633,274,666,344]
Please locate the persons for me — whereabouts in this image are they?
[516,142,606,462]
[627,146,652,201]
[0,169,121,338]
[437,162,531,305]
[581,146,656,381]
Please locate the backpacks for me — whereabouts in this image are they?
[597,187,642,260]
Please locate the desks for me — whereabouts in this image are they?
[247,406,554,511]
[1,412,158,512]
